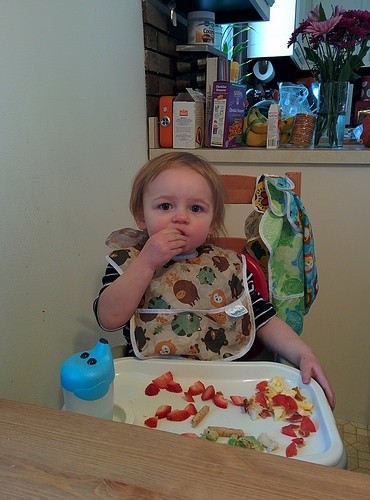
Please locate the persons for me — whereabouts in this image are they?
[93,152,336,412]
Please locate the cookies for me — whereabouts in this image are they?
[291,113,317,146]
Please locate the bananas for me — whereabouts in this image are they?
[243,107,295,146]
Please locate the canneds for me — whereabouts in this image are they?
[187,10,215,46]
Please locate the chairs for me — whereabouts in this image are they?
[62,170,348,469]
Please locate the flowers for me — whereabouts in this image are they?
[289,1,370,149]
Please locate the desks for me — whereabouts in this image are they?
[1,397,370,500]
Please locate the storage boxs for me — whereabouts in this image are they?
[158,80,247,149]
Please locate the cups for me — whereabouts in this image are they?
[60,338,115,422]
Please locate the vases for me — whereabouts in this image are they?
[316,83,348,149]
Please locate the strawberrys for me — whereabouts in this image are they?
[144,371,316,457]
